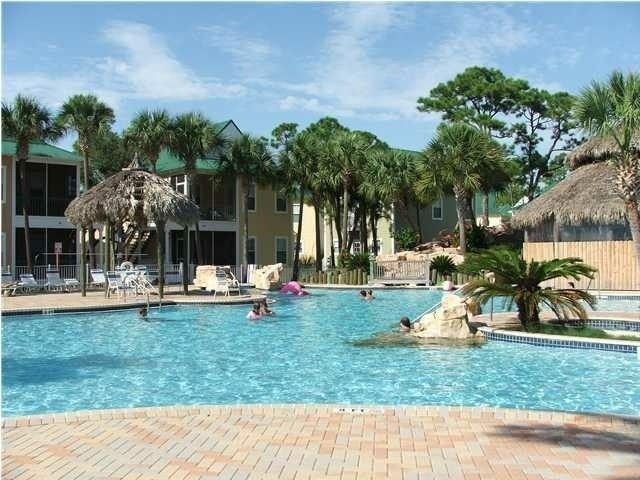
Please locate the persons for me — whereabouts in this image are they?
[139,305,147,318]
[367,290,373,301]
[360,290,369,303]
[392,317,411,332]
[246,302,260,320]
[258,297,272,316]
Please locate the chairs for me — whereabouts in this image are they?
[1,258,240,308]
